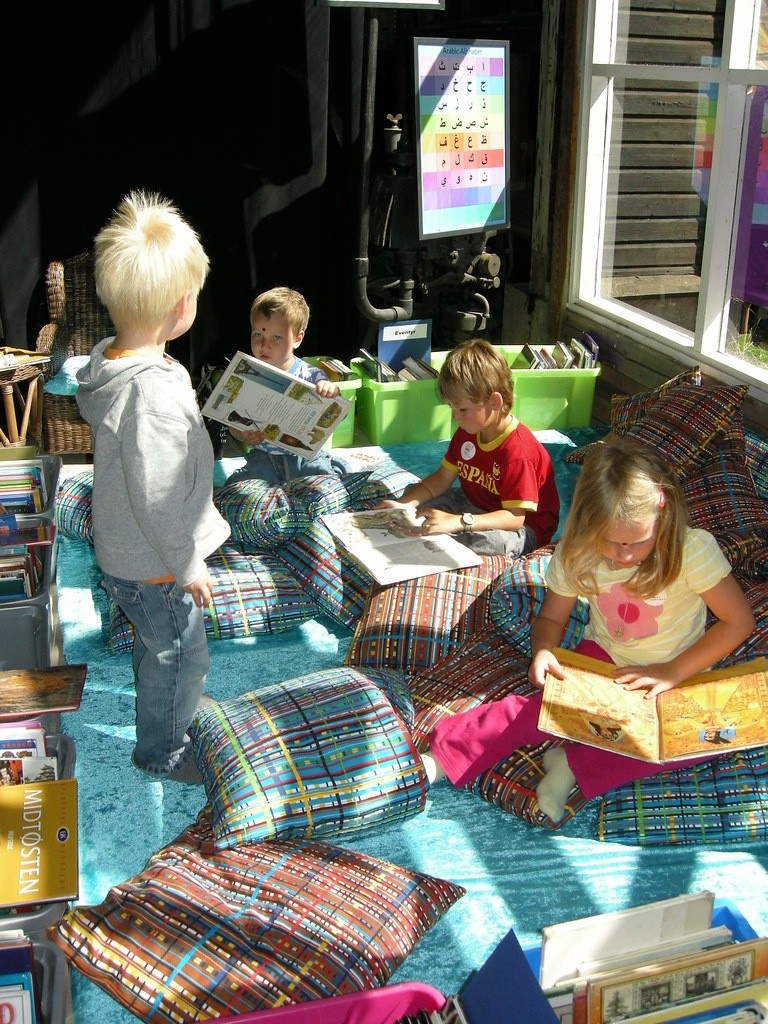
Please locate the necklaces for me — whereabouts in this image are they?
[602,597,630,637]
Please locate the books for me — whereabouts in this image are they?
[318,504,484,586]
[0,445,57,603]
[201,350,354,459]
[396,888,768,1024]
[510,330,599,369]
[0,720,79,914]
[360,347,439,382]
[0,928,43,1024]
[536,646,768,765]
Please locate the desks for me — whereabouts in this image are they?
[0,361,49,453]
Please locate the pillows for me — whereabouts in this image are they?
[55,469,98,544]
[48,795,469,1024]
[210,469,375,551]
[186,661,431,851]
[111,543,323,656]
[273,366,768,850]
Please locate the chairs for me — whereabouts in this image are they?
[35,255,120,455]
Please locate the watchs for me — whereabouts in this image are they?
[462,512,474,531]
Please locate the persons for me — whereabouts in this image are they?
[373,338,560,557]
[222,287,352,494]
[420,435,757,824]
[74,188,230,786]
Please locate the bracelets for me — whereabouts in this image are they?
[420,483,434,500]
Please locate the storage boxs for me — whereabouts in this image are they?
[490,344,602,431]
[208,354,361,459]
[192,981,448,1024]
[0,452,75,1024]
[350,347,461,448]
[522,896,760,1015]
